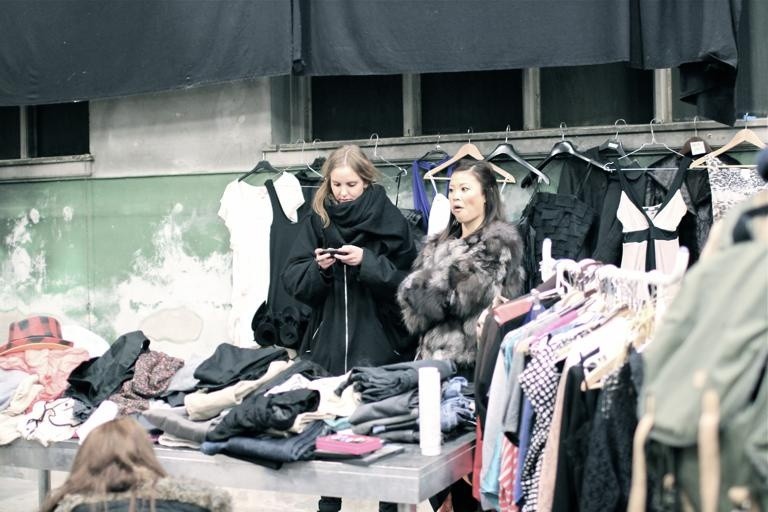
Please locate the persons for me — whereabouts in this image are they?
[38,415,232,511]
[281,142,415,511]
[398,160,527,510]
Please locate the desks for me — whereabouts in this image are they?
[1,396,482,511]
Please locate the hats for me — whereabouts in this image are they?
[0,316,73,355]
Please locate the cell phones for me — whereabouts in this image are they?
[320,250,348,258]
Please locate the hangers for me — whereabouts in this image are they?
[495,257,673,337]
[235,109,768,186]
